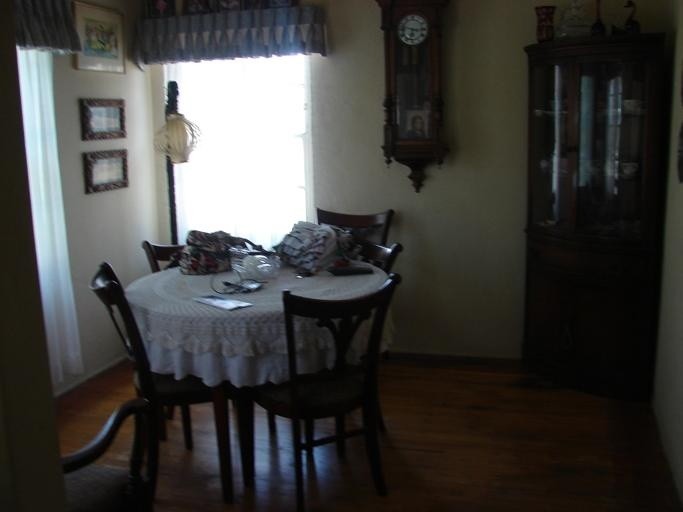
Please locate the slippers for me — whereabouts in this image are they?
[274,220,364,275]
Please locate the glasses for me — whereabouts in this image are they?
[151,81,204,245]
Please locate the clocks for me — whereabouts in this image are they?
[375,0,450,193]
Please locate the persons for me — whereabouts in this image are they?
[407,116,425,137]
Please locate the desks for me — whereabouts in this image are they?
[126,262,389,479]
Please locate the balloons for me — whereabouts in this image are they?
[375,0,450,193]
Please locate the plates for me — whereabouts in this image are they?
[519,43,676,401]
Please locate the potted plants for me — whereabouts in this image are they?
[79,97,130,194]
[70,0,126,74]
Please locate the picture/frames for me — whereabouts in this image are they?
[70,0,126,74]
[79,97,130,194]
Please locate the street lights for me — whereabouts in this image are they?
[179,230,249,275]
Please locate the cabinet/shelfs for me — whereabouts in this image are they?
[519,43,676,401]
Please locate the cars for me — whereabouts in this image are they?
[620,162,638,176]
[622,99,644,113]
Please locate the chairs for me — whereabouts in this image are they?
[87,261,239,505]
[143,209,402,295]
[239,273,402,508]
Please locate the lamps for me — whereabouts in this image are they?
[151,81,204,245]
